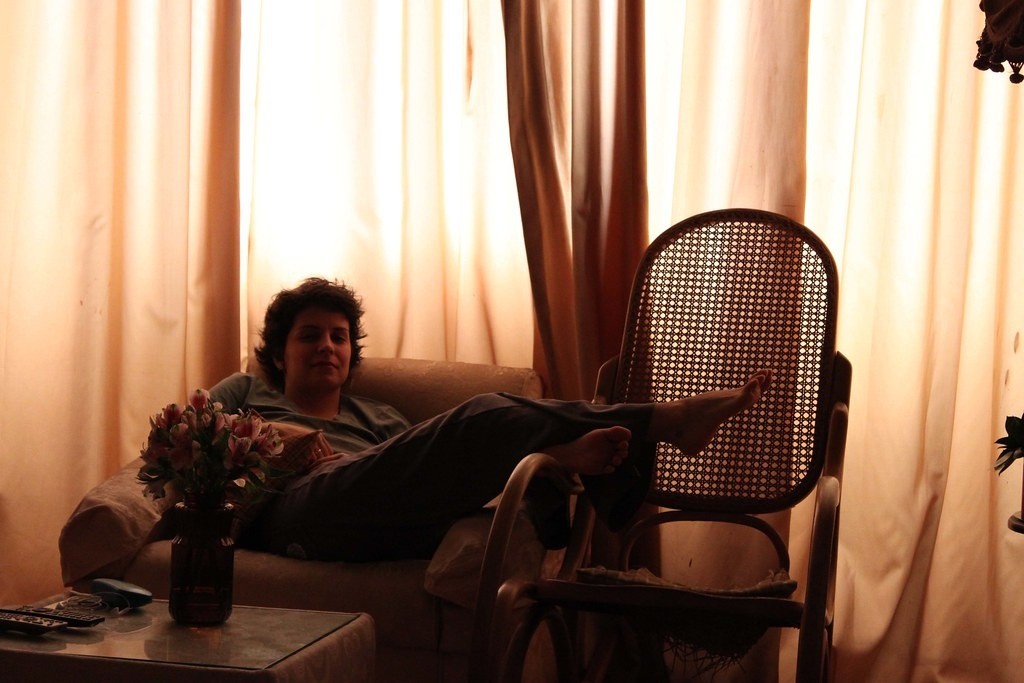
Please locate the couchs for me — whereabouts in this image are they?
[60,350,545,682]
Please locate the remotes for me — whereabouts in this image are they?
[0,601,105,635]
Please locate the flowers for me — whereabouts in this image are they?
[137,385,285,505]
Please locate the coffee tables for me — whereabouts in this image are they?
[0,595,377,683]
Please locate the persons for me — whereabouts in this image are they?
[191,277,773,563]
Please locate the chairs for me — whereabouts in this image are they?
[466,207,852,683]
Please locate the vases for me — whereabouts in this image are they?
[166,509,236,624]
